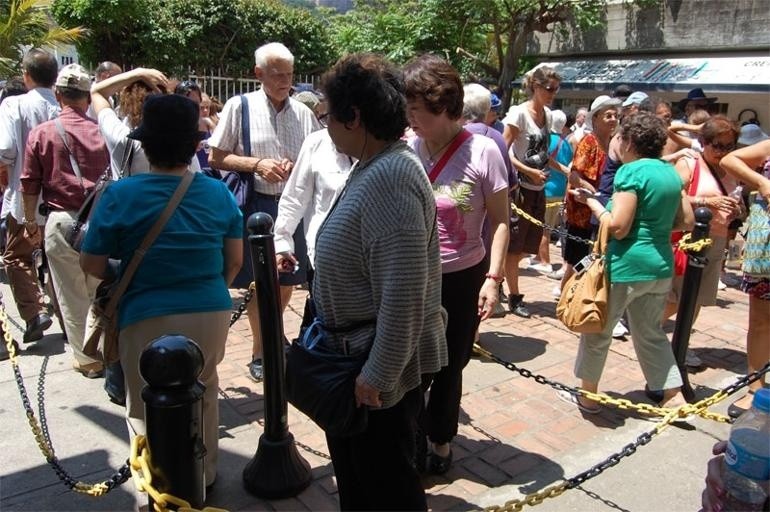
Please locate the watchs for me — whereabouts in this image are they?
[20,216,42,226]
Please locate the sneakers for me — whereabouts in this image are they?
[685,348,701,368]
[612,320,628,338]
[529,259,553,274]
[249,359,262,382]
[74,362,103,378]
[548,270,567,279]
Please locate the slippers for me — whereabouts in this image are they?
[554,388,602,415]
[641,402,696,423]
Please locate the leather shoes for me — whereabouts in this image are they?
[640,382,697,403]
[728,390,758,416]
[22,314,53,345]
[429,447,452,471]
[509,293,530,317]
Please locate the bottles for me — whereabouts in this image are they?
[715,186,743,224]
[720,388,770,511]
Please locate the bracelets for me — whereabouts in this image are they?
[252,157,265,174]
[24,224,39,242]
[596,209,608,221]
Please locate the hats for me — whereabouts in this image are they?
[53,62,91,96]
[489,94,502,107]
[622,91,649,107]
[736,124,770,144]
[128,95,212,142]
[678,88,717,109]
[586,95,622,116]
[611,84,633,95]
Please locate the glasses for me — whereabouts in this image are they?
[536,83,561,92]
[317,108,350,125]
[708,137,734,152]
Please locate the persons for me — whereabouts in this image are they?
[697,439,769,512]
[0,46,68,344]
[589,95,702,256]
[564,113,575,130]
[90,65,172,179]
[621,89,649,120]
[524,108,573,274]
[661,113,743,369]
[84,61,123,121]
[195,91,218,170]
[718,171,749,291]
[555,110,696,424]
[719,138,770,419]
[498,65,564,318]
[19,63,113,379]
[312,54,448,512]
[560,94,623,296]
[206,41,325,384]
[487,93,505,136]
[401,55,511,476]
[461,83,519,227]
[569,107,590,133]
[273,128,360,271]
[79,93,245,497]
[667,109,710,153]
[678,86,718,125]
[174,80,202,105]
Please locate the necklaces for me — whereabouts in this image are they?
[424,127,465,167]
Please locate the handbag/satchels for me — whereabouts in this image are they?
[66,127,134,257]
[555,213,613,334]
[670,156,701,276]
[742,192,770,278]
[285,289,363,431]
[220,90,258,207]
[519,150,548,176]
[82,168,195,364]
[495,308,498,309]
